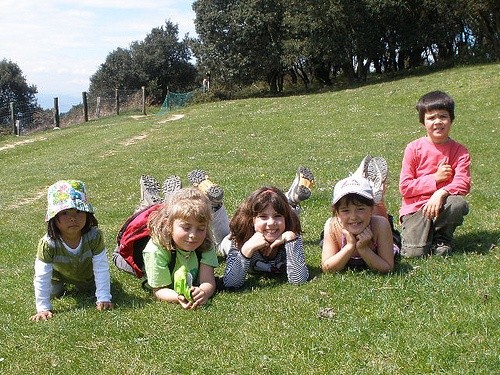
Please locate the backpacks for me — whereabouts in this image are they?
[113,203,201,276]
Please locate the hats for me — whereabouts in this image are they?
[333,178,373,206]
[45,179,95,221]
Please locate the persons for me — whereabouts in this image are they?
[398,91,471,258]
[28,180,113,323]
[115,174,219,309]
[321,154,399,273]
[189,165,314,286]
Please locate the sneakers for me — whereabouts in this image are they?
[136,175,163,207]
[352,155,388,203]
[187,170,223,206]
[161,175,182,199]
[286,166,314,206]
[434,240,452,257]
